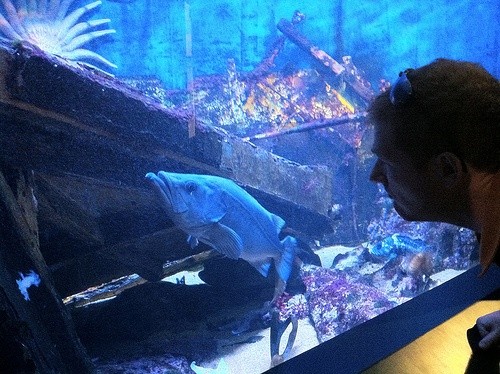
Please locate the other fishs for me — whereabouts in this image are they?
[144,170,297,282]
[11,46,27,86]
[371,234,438,262]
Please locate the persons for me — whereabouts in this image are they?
[366,58,500,374]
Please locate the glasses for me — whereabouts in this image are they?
[388,64,438,162]
